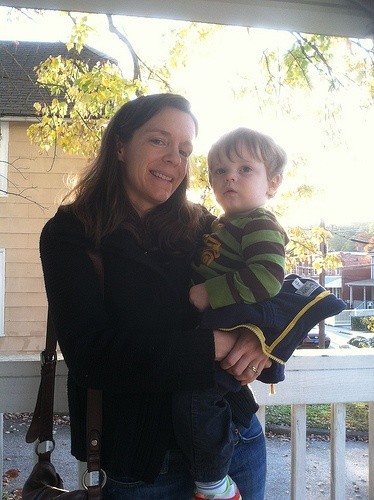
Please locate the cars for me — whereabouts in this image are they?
[298,326,331,348]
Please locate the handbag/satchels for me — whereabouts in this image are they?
[22,462,101,500]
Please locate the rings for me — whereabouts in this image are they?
[248,364,258,373]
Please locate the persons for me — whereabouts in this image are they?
[169,128,289,500]
[40,93,272,500]
[345,299,374,309]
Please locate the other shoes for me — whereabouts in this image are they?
[191,476,242,500]
